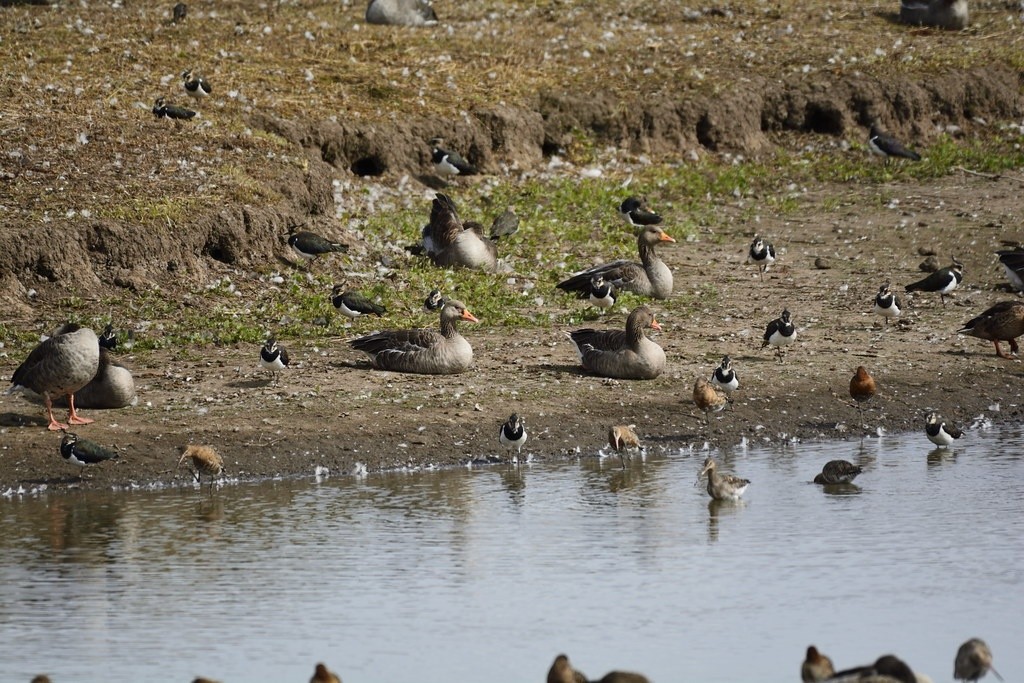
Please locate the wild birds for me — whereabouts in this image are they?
[178,444,223,487]
[151,68,213,121]
[259,337,290,380]
[282,222,350,270]
[9,322,118,477]
[489,197,796,502]
[850,365,876,408]
[546,637,1004,683]
[352,156,385,177]
[60,327,135,409]
[366,0,439,26]
[174,3,187,22]
[924,412,962,448]
[859,113,922,164]
[955,247,1024,358]
[814,459,863,486]
[329,279,478,375]
[874,256,964,324]
[427,139,478,182]
[404,192,498,271]
